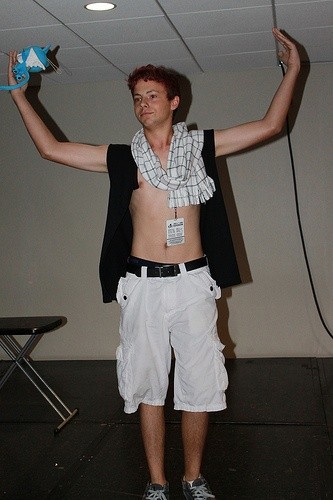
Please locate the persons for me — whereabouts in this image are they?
[7,27,300,500]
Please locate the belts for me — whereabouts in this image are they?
[128,256,207,278]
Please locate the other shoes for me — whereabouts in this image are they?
[142,482,170,500]
[181,475,215,500]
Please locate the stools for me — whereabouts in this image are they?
[0,316,79,435]
